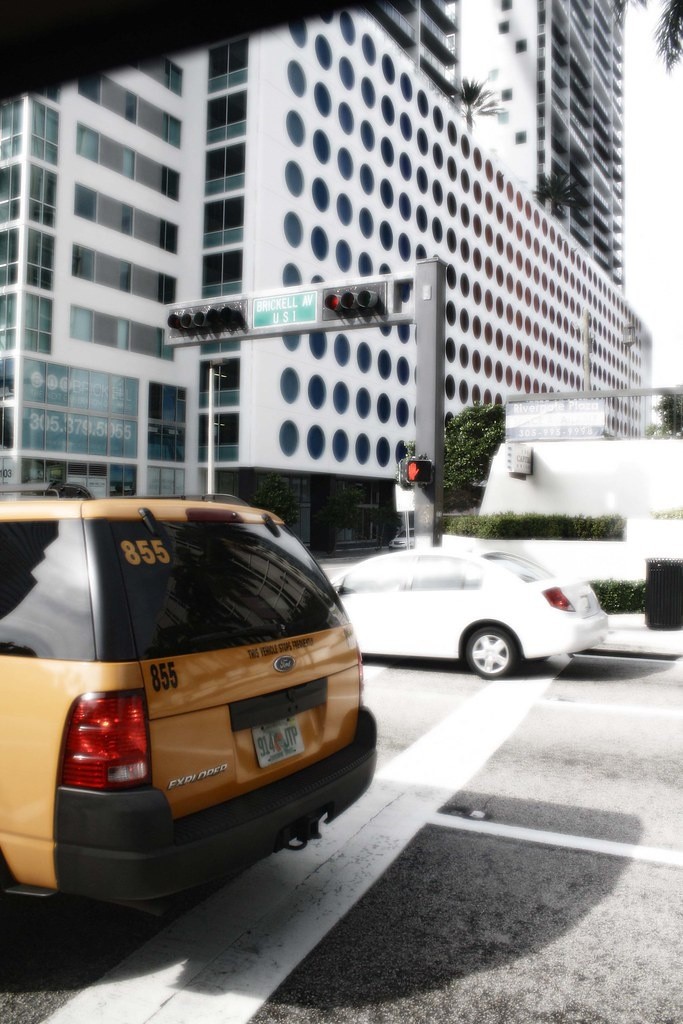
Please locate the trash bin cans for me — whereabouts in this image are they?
[645,558,683,631]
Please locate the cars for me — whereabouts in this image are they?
[389,531,416,550]
[264,546,612,680]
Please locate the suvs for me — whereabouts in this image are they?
[0,484,381,913]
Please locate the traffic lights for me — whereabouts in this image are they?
[405,459,433,487]
[321,281,386,322]
[168,298,243,338]
